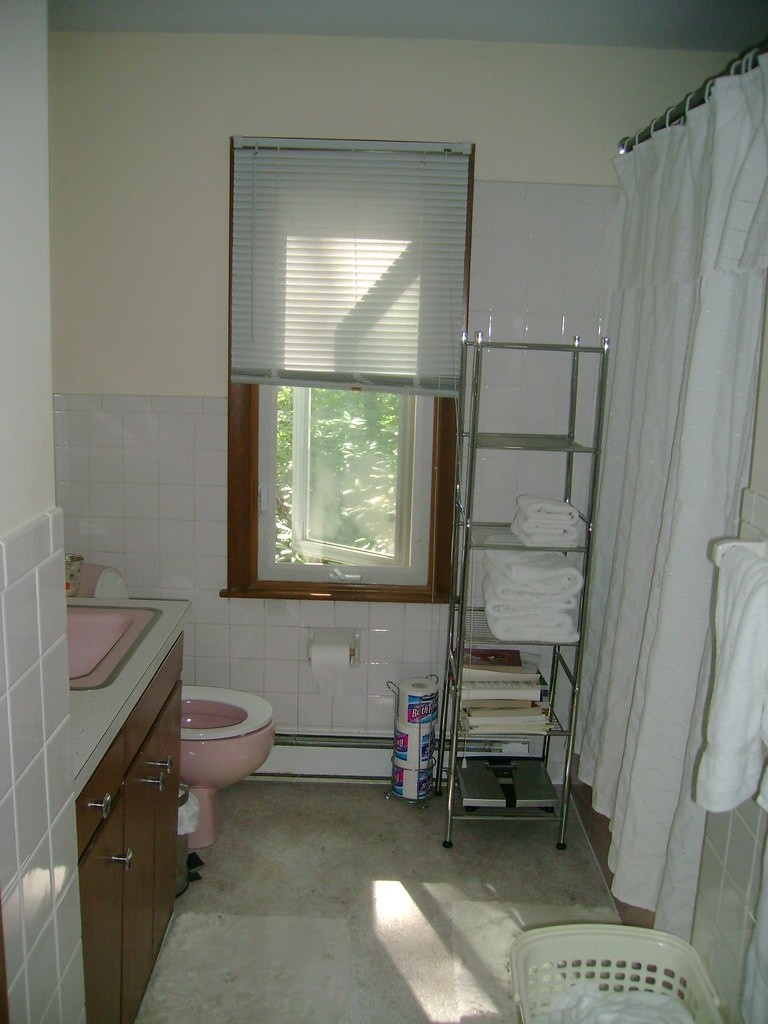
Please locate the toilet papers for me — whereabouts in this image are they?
[393,678,438,800]
[311,643,351,696]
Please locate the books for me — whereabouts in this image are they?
[459,648,548,736]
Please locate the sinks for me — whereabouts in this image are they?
[66,604,163,691]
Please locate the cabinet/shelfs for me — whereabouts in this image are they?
[121,679,178,1024]
[434,339,609,847]
[79,730,121,1024]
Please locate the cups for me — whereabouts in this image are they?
[65,554,84,597]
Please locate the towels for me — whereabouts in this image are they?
[535,979,695,1024]
[693,547,768,813]
[481,533,584,642]
[510,494,579,548]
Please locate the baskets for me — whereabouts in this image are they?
[510,923,724,1024]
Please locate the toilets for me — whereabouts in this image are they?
[72,563,276,850]
